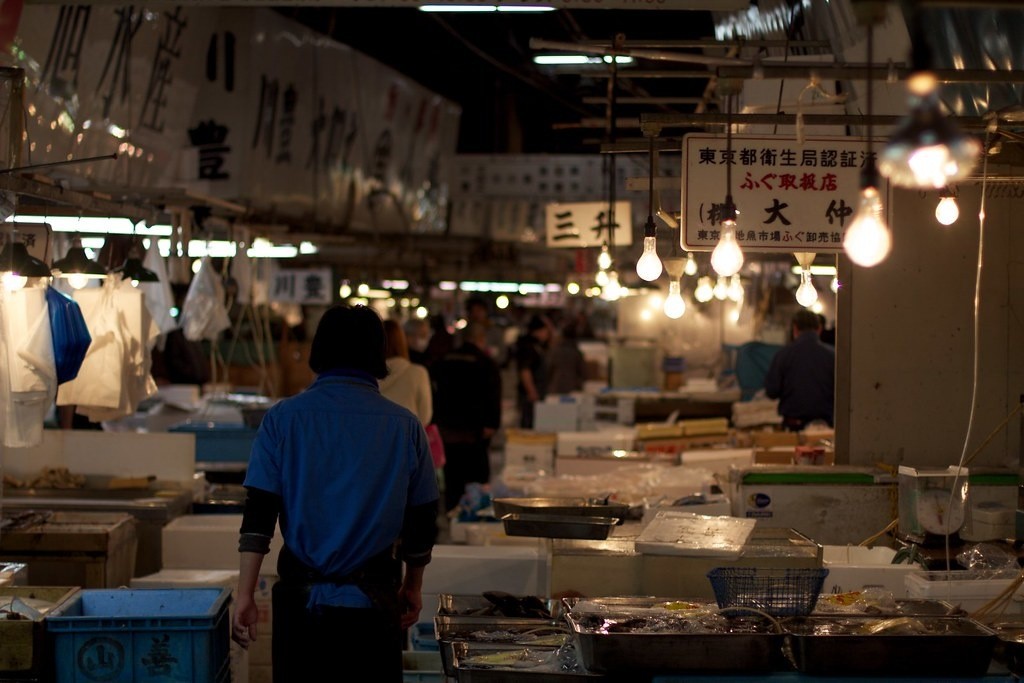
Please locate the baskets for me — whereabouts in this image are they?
[706,566,831,616]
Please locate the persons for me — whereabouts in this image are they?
[231,296,592,683]
[767,310,835,432]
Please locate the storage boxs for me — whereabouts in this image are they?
[0,380,1015,683]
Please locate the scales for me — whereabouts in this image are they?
[899,465,973,546]
